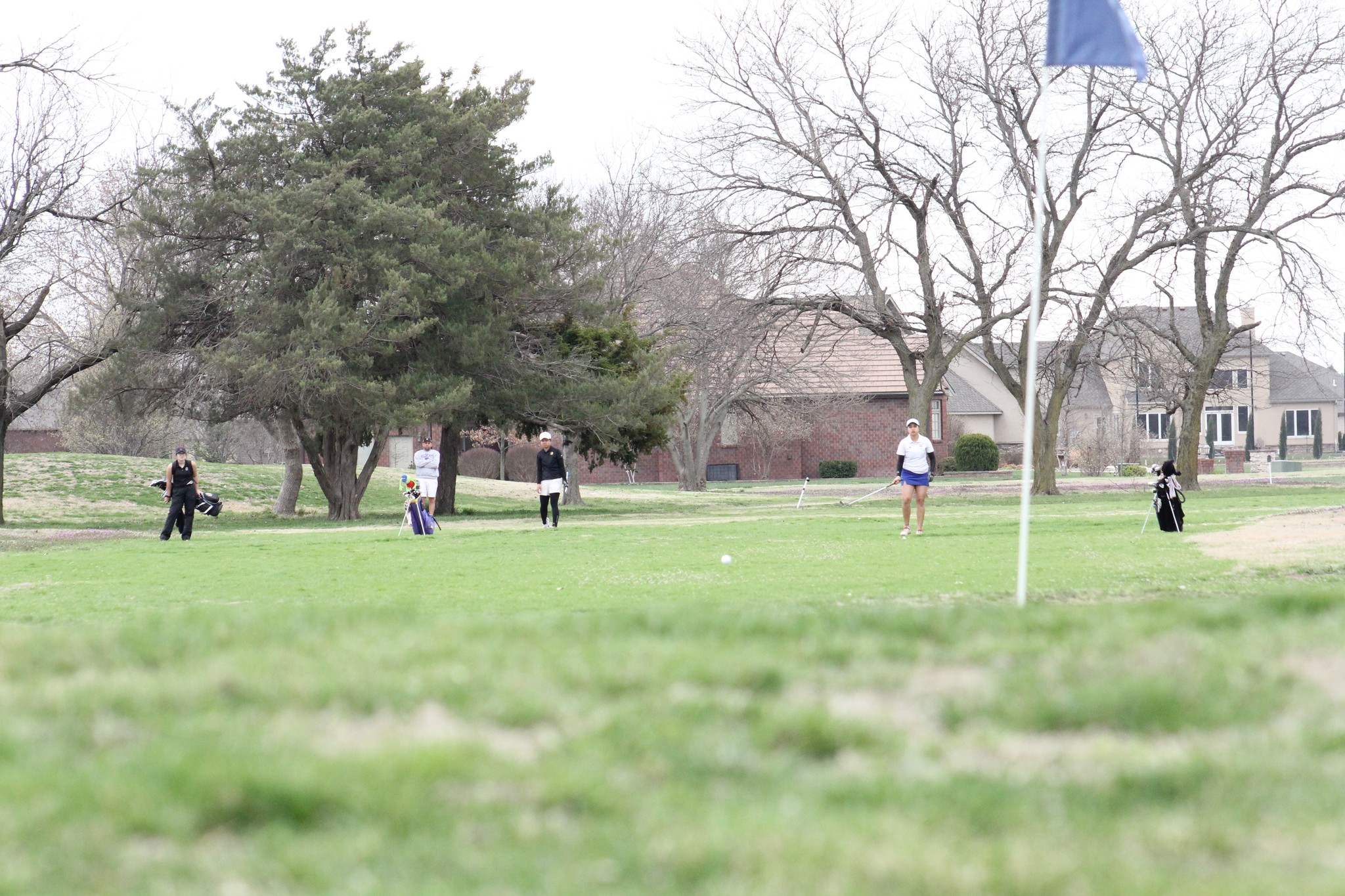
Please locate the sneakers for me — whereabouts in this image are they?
[542,517,550,528]
[900,526,910,535]
[182,536,190,541]
[916,530,922,534]
[160,534,168,541]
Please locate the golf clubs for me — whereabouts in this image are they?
[402,488,420,500]
[1147,481,1160,487]
[839,478,904,507]
[149,478,168,497]
[1148,463,1161,476]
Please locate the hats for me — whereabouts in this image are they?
[906,419,920,427]
[423,437,432,443]
[176,447,187,455]
[539,432,551,441]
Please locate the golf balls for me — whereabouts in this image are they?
[721,554,731,565]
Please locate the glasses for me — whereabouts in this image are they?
[177,452,186,455]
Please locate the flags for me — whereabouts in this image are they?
[1046,0,1146,82]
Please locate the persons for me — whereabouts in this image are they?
[160,447,203,541]
[537,432,569,528]
[414,437,440,518]
[892,418,936,536]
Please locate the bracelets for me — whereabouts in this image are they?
[165,495,171,498]
